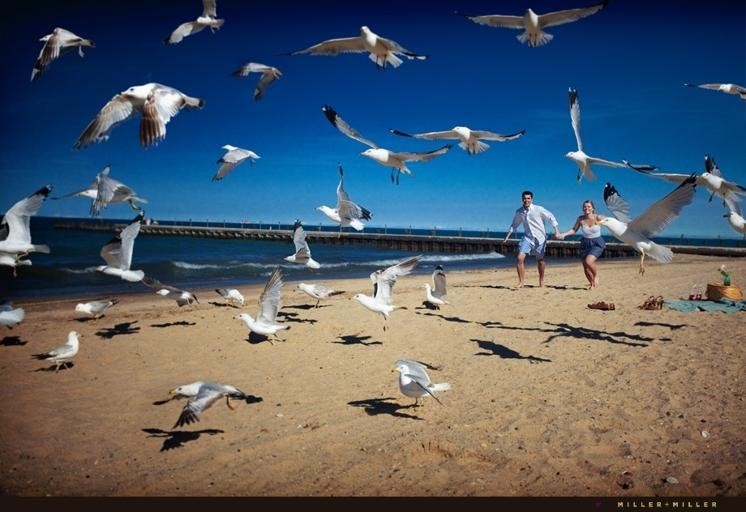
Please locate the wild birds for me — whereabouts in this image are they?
[0,167,199,372]
[168,1,225,45]
[153,381,263,431]
[564,84,746,276]
[466,5,602,48]
[227,62,283,101]
[30,27,96,83]
[392,359,453,407]
[72,83,205,151]
[287,26,431,73]
[215,105,527,346]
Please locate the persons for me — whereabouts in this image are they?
[559,199,605,291]
[501,191,560,289]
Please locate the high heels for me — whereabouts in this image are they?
[639,296,664,310]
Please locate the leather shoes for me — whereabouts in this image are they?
[588,301,615,310]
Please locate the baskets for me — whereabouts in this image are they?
[707,282,744,302]
